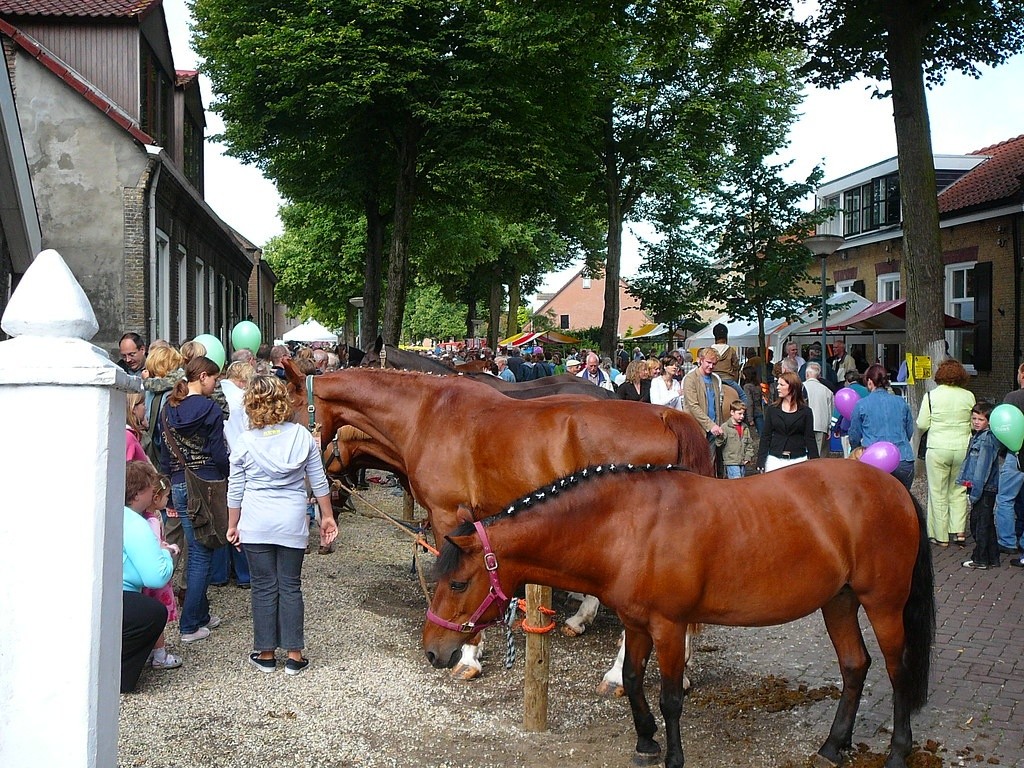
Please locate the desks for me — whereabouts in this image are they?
[889,381,908,404]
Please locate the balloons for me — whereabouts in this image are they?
[860,441,901,473]
[232,321,262,355]
[193,334,226,373]
[989,404,1024,452]
[834,387,861,421]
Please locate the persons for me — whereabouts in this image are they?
[615,323,1024,570]
[116,332,339,693]
[410,345,621,392]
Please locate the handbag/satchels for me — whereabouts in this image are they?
[917,430,928,460]
[184,467,228,549]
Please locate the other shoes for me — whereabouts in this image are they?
[286,656,310,676]
[953,533,965,542]
[962,560,987,569]
[202,618,220,628]
[989,561,999,567]
[249,653,276,673]
[319,542,337,554]
[181,628,209,641]
[152,653,182,668]
[930,538,949,546]
[999,544,1018,554]
[1010,559,1024,566]
[304,544,309,552]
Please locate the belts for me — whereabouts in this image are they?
[780,453,792,459]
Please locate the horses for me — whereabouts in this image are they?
[280,334,716,700]
[422,458,939,768]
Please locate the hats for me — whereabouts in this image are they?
[566,359,581,366]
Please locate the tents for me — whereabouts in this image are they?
[624,323,695,341]
[499,330,582,358]
[283,317,338,341]
[685,291,973,366]
